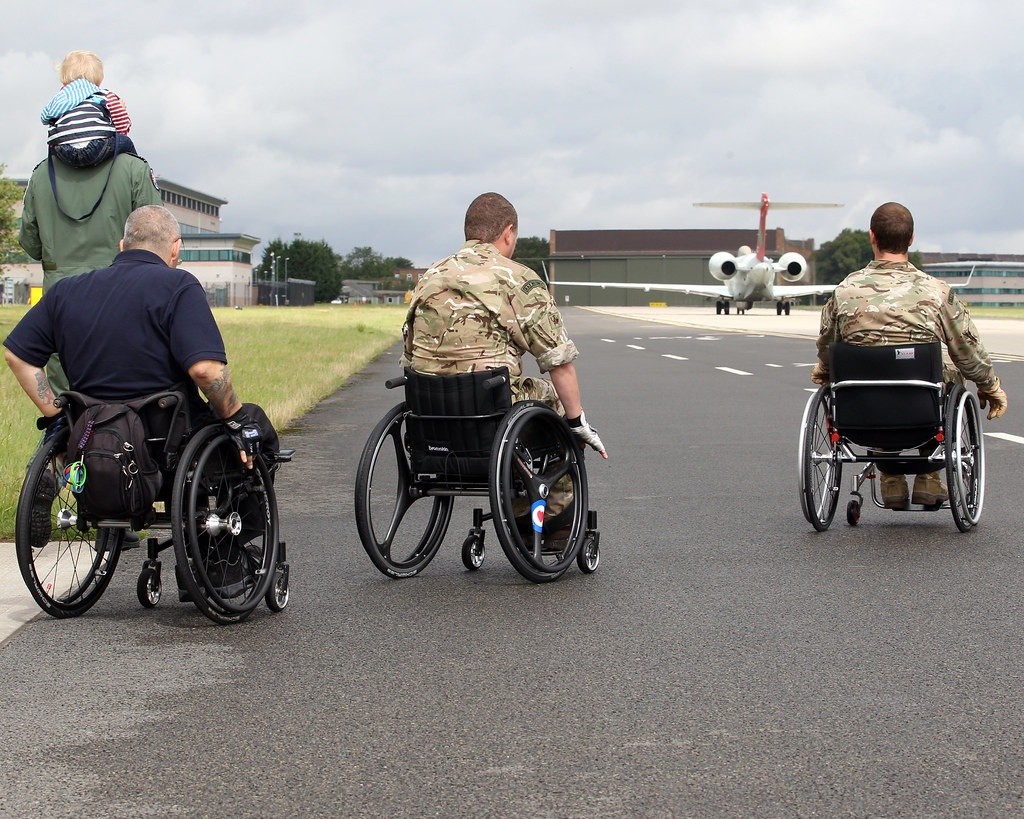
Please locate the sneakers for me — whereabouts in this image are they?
[911,474,949,504]
[95,528,140,550]
[880,474,909,508]
[522,522,571,553]
[175,545,262,602]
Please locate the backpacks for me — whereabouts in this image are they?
[59,391,192,532]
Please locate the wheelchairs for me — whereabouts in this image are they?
[355,363,601,585]
[797,336,986,534]
[14,376,297,625]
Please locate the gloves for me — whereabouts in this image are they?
[977,376,1007,420]
[811,362,829,385]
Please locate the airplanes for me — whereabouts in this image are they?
[541,192,976,314]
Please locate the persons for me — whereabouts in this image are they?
[17,49,164,551]
[3,204,281,601]
[399,193,608,550]
[811,202,1007,509]
